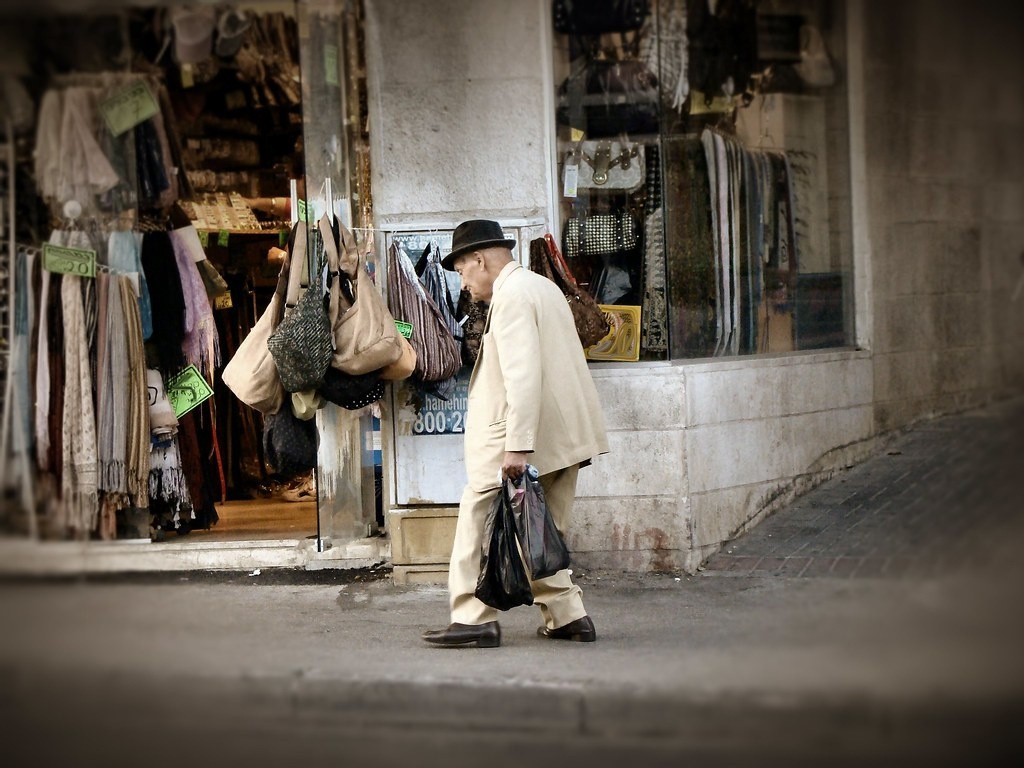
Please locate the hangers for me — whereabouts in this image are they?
[53,215,170,239]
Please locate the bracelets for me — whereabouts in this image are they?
[270,197,276,213]
[278,251,287,263]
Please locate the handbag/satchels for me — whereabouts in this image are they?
[555,1,832,134]
[563,193,637,258]
[220,211,492,421]
[584,252,641,362]
[530,233,611,349]
[475,463,570,610]
[561,131,647,196]
[642,290,713,352]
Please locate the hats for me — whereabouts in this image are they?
[441,218,516,272]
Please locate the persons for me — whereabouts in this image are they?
[243,137,316,502]
[420,219,611,646]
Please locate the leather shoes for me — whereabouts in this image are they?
[537,614,596,641]
[422,621,501,648]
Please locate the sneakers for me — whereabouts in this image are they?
[281,476,318,502]
